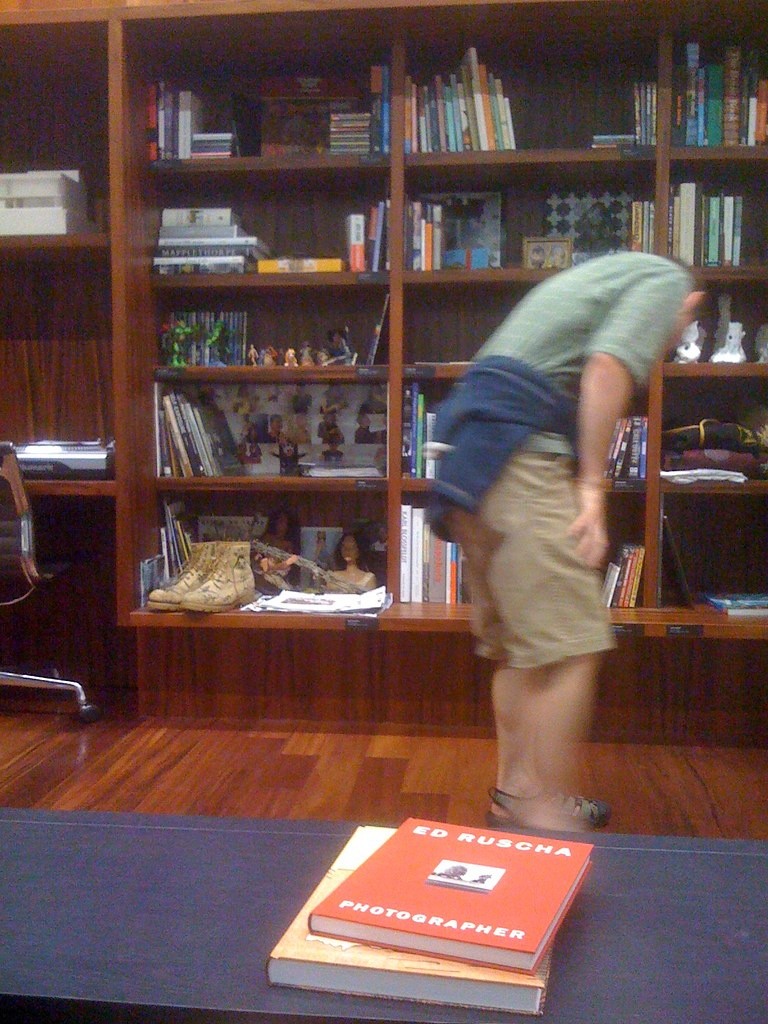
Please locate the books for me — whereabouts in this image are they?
[369,63,391,156]
[154,382,243,478]
[405,47,516,155]
[401,382,438,480]
[264,824,554,1015]
[404,194,442,272]
[153,207,270,274]
[158,308,248,367]
[590,133,635,149]
[310,461,383,478]
[146,80,234,160]
[258,258,342,273]
[670,40,768,148]
[308,816,595,976]
[669,182,742,267]
[624,79,657,146]
[630,200,655,255]
[345,199,390,273]
[328,111,370,155]
[600,414,768,617]
[14,439,109,470]
[400,504,464,604]
[140,501,195,608]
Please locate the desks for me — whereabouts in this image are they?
[1,806,768,1024]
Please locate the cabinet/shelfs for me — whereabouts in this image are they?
[0,0,768,744]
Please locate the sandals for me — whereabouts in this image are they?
[484,786,609,831]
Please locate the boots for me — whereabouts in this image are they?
[147,542,216,612]
[180,540,255,612]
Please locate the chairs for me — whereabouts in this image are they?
[1,437,103,725]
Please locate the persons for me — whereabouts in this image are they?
[324,528,377,594]
[253,509,297,595]
[242,320,360,365]
[423,250,703,832]
